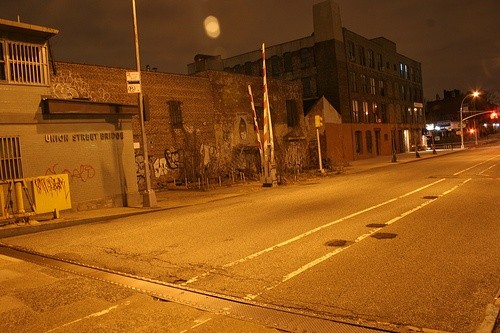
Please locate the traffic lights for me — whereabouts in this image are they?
[314,115,323,129]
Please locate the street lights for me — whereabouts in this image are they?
[460,92,480,150]
